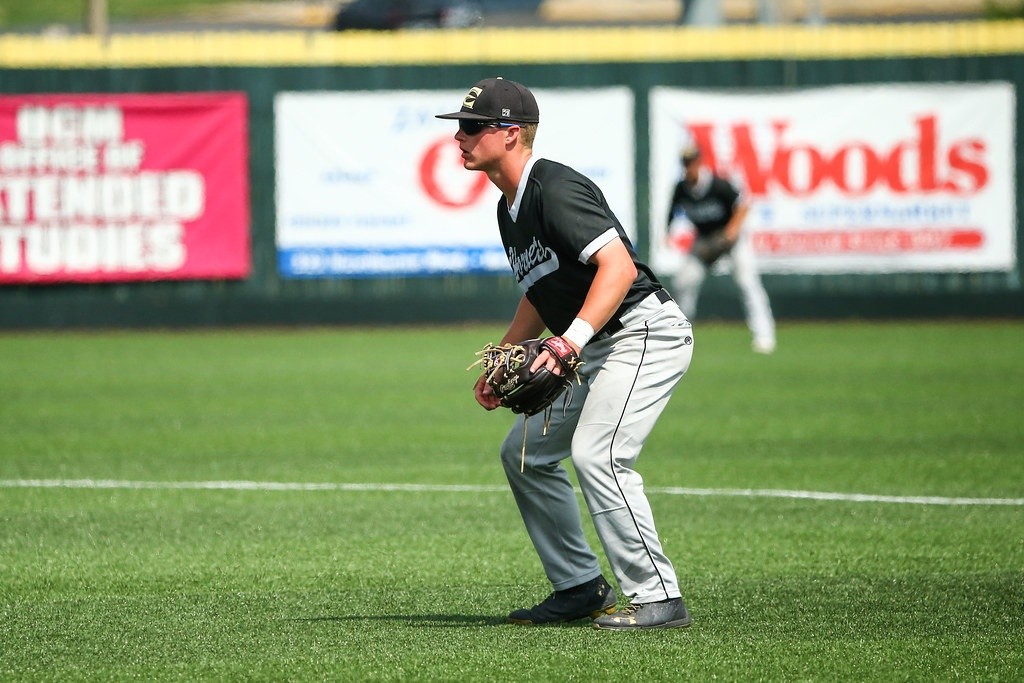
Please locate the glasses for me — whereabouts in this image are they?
[457,119,528,136]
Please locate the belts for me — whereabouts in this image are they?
[586,290,673,345]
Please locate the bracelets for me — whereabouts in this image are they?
[564,317,595,349]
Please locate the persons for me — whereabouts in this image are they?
[434,78,694,631]
[663,145,777,354]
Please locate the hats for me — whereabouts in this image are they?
[435,76,540,126]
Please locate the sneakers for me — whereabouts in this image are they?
[593,593,690,632]
[509,572,618,626]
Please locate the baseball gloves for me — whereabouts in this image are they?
[692,234,734,267]
[485,333,584,417]
